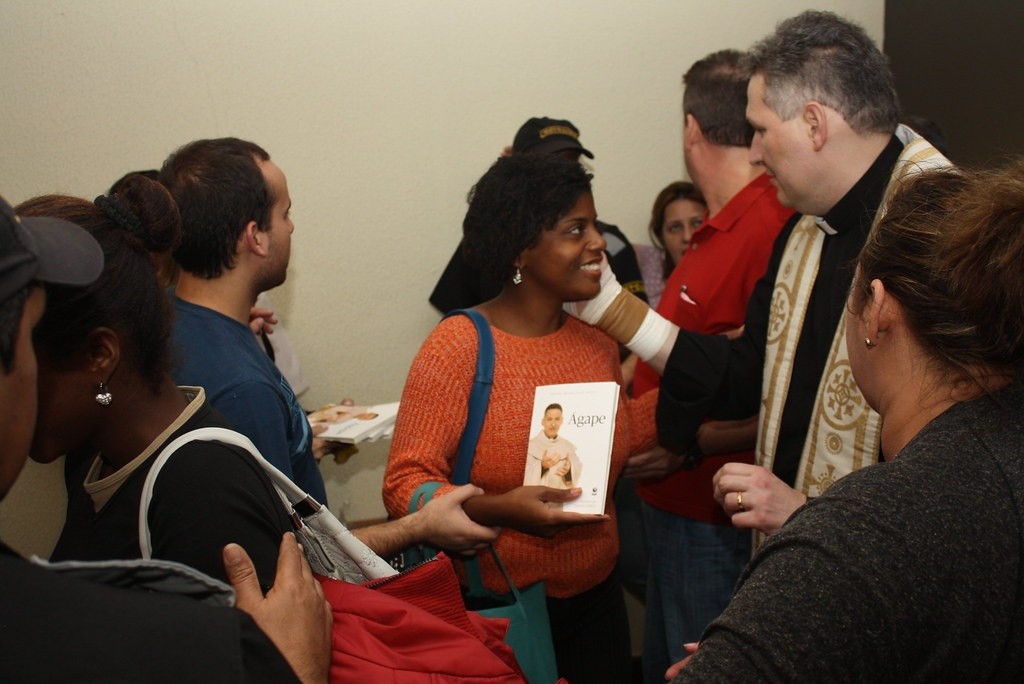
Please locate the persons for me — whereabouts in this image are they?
[650,181,705,274]
[667,169,1024,684]
[252,293,355,463]
[563,12,962,557]
[383,136,745,684]
[511,116,650,381]
[333,406,379,422]
[163,138,505,556]
[0,169,361,684]
[627,48,799,684]
[524,404,583,511]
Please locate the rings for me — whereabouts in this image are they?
[737,491,744,509]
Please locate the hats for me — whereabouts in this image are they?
[512,117,595,159]
[0,195,105,300]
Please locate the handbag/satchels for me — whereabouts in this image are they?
[140,427,401,583]
[404,480,559,684]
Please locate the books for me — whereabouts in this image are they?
[310,401,400,443]
[523,381,619,516]
